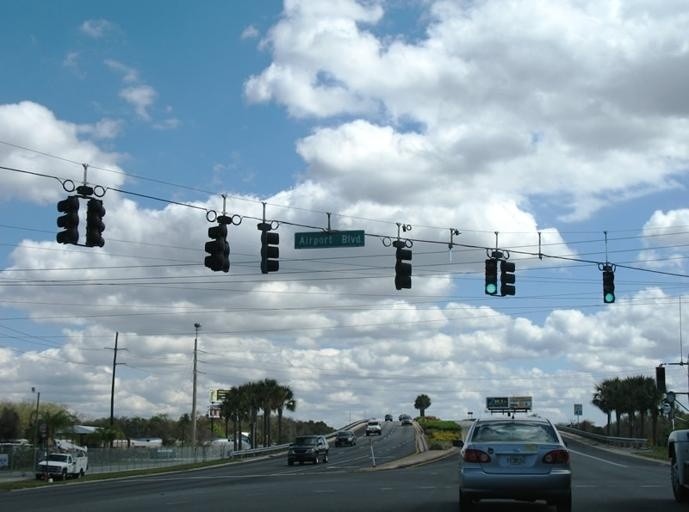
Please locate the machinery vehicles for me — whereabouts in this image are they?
[36,439,88,480]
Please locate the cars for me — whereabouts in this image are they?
[335,431,356,447]
[457,415,572,512]
[385,414,412,425]
[366,421,382,436]
[287,435,329,466]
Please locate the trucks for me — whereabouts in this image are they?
[656,356,689,503]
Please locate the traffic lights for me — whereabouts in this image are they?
[394,248,412,291]
[485,259,516,297]
[602,270,616,303]
[260,232,279,275]
[205,224,230,273]
[56,195,105,247]
